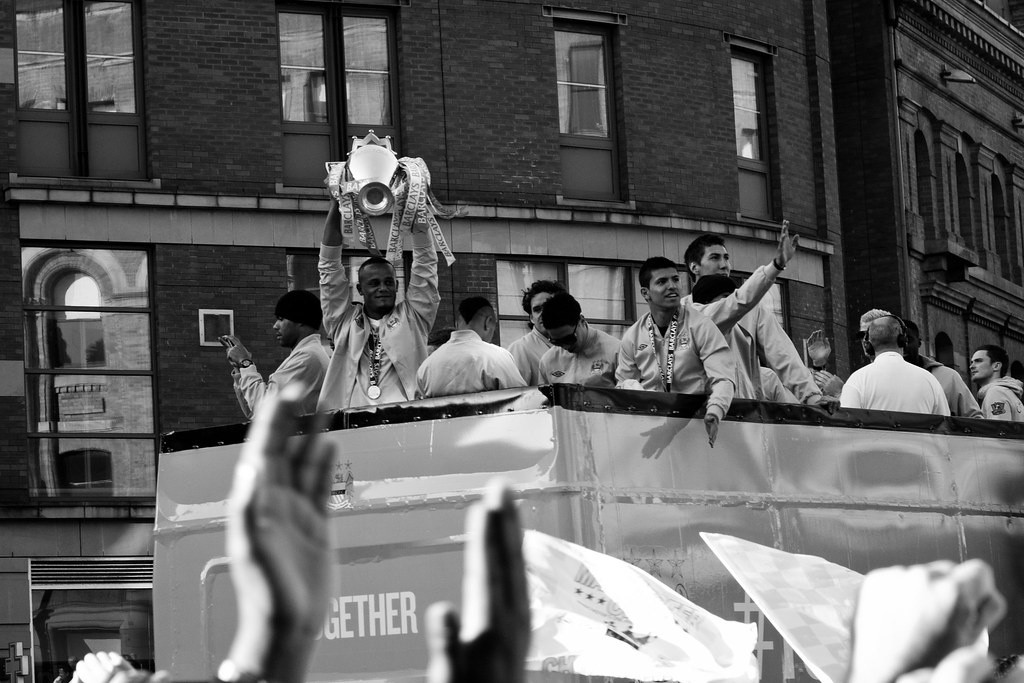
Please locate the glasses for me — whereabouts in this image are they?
[549,323,578,347]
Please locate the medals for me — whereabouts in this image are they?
[366,385,380,399]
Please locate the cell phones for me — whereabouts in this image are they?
[226,338,233,348]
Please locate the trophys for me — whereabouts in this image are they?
[324,129,409,215]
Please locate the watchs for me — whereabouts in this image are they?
[237,359,254,369]
[774,257,787,270]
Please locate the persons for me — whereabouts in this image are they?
[640,393,712,459]
[314,173,440,415]
[614,255,738,449]
[54,651,161,683]
[216,289,330,419]
[213,387,531,683]
[413,204,1024,421]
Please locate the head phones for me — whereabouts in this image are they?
[863,315,911,356]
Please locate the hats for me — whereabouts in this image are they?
[275,290,323,331]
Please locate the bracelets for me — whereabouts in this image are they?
[812,362,828,371]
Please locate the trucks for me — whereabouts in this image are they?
[152,382,1023,682]
